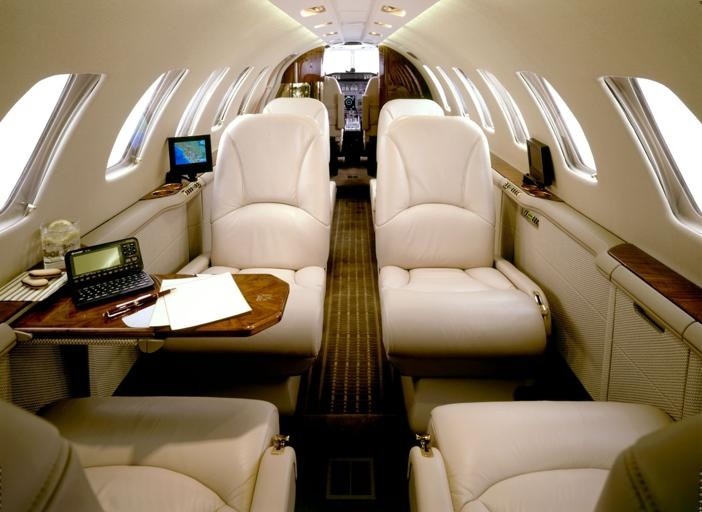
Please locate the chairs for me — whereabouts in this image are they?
[408,397,701,512]
[0,397,298,512]
[362,76,378,149]
[137,112,330,412]
[325,77,345,152]
[264,97,328,113]
[376,99,445,162]
[371,117,554,432]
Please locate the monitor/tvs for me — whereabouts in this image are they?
[526,137,553,187]
[168,134,213,181]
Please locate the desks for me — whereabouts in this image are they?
[13,265,290,347]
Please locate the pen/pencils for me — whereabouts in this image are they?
[102,287,177,318]
